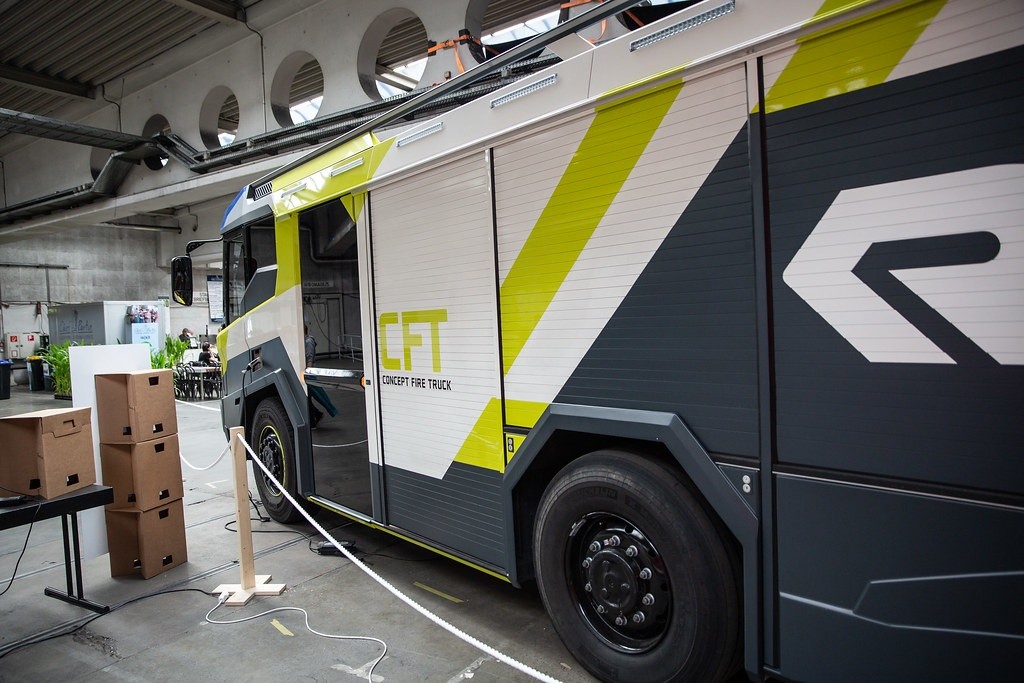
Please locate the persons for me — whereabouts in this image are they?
[199,342,213,393]
[304,321,324,431]
[179,328,191,349]
[130,307,158,323]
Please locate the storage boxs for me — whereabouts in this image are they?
[95,369,178,445]
[0,407,96,499]
[104,498,188,581]
[99,434,184,513]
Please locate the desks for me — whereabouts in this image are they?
[0,485,113,614]
[171,366,222,401]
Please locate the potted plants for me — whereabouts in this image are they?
[40,340,73,401]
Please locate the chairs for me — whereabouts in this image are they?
[176,357,222,402]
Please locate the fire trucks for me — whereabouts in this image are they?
[167,1,1024,681]
